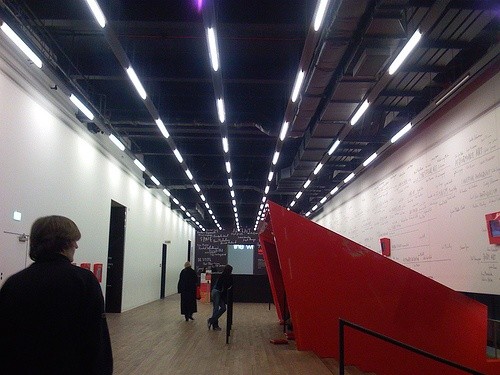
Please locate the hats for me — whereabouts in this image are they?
[184,262,191,268]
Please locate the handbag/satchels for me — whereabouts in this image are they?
[196,286,200,298]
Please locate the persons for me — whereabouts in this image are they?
[177,262,200,321]
[1,215,114,375]
[206,264,233,330]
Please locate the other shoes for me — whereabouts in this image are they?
[213,326,221,330]
[189,316,194,320]
[186,318,188,321]
[208,318,212,330]
